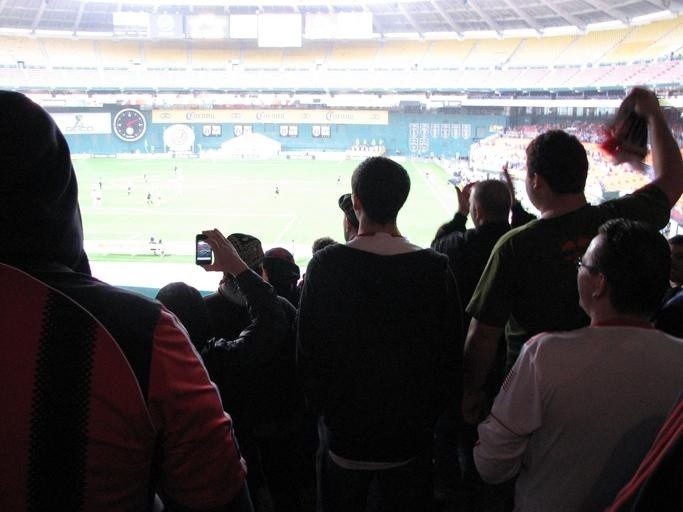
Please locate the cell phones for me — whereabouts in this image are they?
[195,233,212,265]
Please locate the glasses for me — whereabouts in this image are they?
[575,257,607,281]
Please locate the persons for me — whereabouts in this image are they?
[293,154,467,511]
[147,194,359,512]
[472,215,682,512]
[0,89,255,509]
[652,234,683,339]
[335,174,342,187]
[272,184,280,199]
[462,84,683,509]
[424,169,429,180]
[429,156,539,344]
[85,144,177,258]
[440,116,681,218]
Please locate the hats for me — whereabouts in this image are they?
[264,248,295,273]
[227,234,264,268]
[339,194,358,218]
[0,90,76,232]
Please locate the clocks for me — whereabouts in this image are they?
[113,108,147,143]
